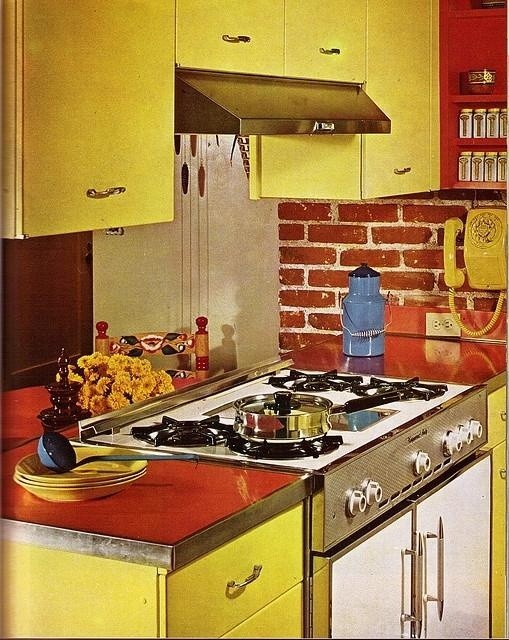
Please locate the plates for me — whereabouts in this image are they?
[13,445,149,503]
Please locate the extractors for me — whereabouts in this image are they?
[174,65,392,136]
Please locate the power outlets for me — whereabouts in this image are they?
[424,310,462,339]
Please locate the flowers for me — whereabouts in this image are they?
[57,350,176,412]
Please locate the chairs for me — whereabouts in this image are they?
[91,315,210,381]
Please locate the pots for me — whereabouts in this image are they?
[233,390,333,440]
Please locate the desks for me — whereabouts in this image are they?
[1,376,205,456]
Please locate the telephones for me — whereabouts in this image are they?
[444,207,507,291]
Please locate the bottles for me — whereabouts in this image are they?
[458,108,507,139]
[340,262,391,358]
[458,151,507,182]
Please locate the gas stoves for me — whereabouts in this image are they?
[79,355,490,554]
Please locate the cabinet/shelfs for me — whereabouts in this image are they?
[177,3,368,84]
[2,1,175,243]
[158,500,307,639]
[331,452,491,638]
[247,1,439,200]
[442,6,509,191]
[89,132,279,372]
